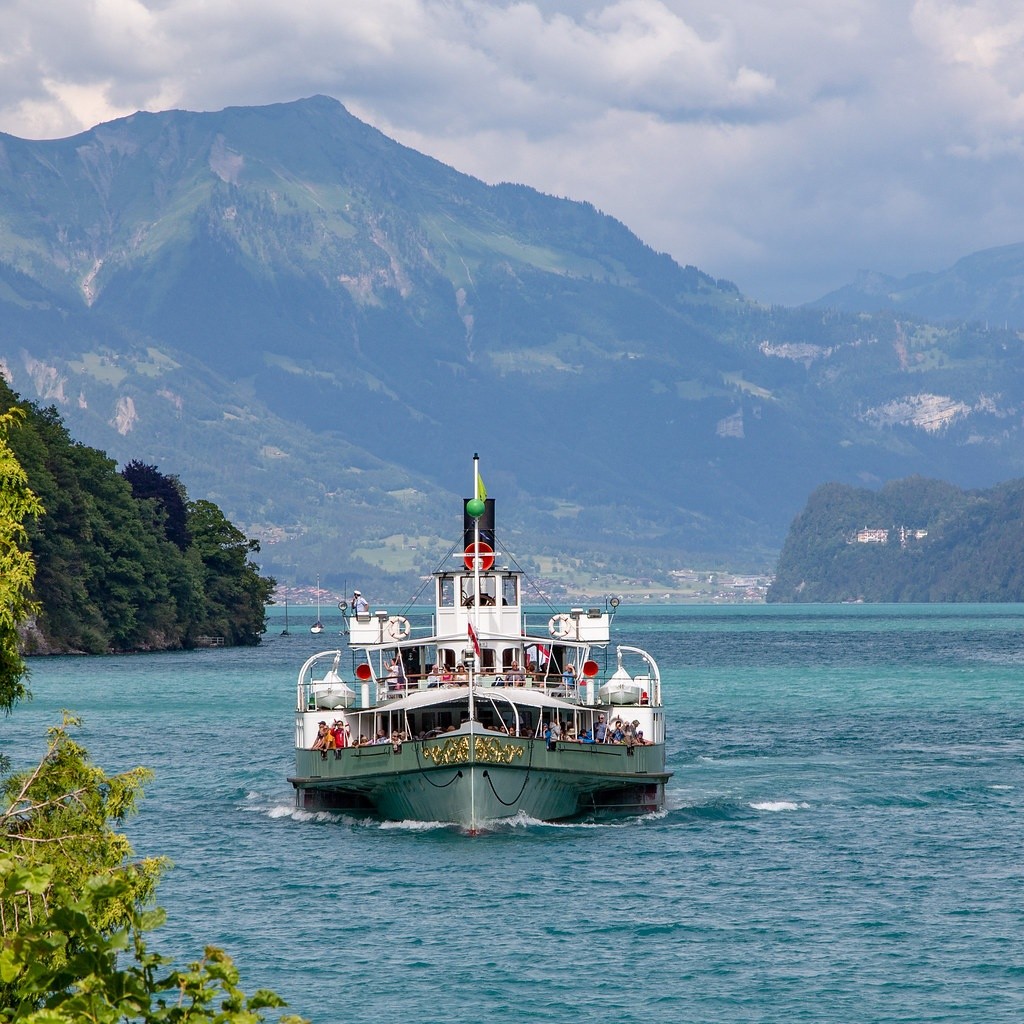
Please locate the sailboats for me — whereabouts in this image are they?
[310,572,325,633]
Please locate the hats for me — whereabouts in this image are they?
[638,731,643,734]
[542,723,548,727]
[354,590,361,596]
[318,721,326,725]
[580,729,589,733]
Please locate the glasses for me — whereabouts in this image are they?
[513,665,517,667]
[598,717,604,720]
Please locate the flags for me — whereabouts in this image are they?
[478,474,487,502]
[467,623,480,657]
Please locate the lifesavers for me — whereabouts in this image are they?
[388,616,412,640]
[548,613,572,638]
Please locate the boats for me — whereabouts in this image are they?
[287,453,674,838]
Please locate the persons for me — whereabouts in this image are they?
[352,591,368,618]
[309,713,652,752]
[383,654,577,691]
